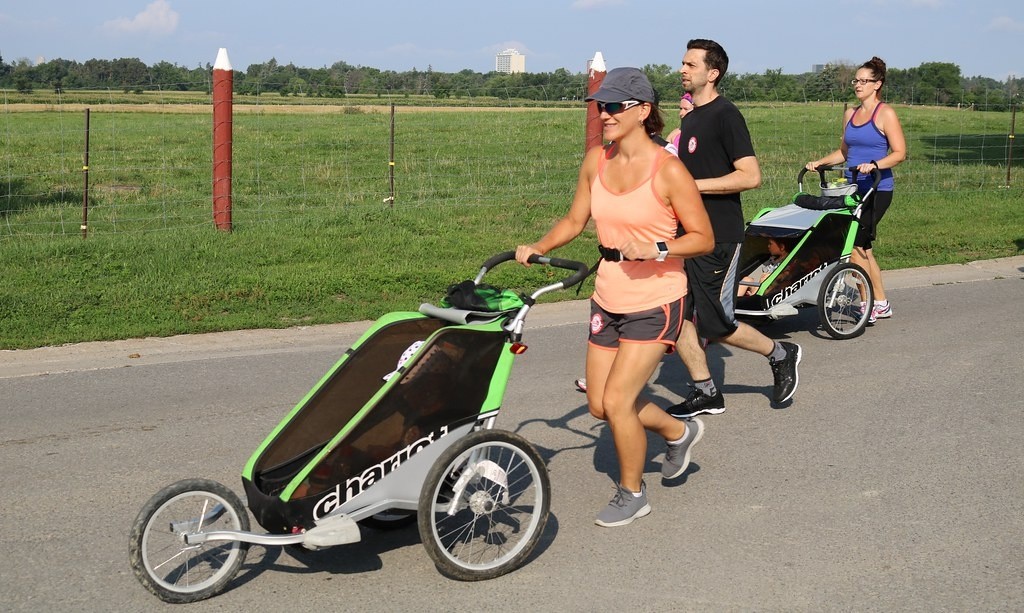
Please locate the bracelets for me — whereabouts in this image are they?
[870,160,879,170]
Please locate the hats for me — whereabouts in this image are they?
[584,67,655,104]
[682,93,695,106]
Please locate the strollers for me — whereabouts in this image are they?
[729,165,881,340]
[126,246,587,605]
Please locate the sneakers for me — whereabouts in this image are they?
[661,416,705,479]
[768,340,802,406]
[575,378,587,391]
[860,304,876,323]
[666,382,725,418]
[594,478,651,527]
[873,300,893,318]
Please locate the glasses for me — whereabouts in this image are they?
[851,78,879,85]
[597,100,646,114]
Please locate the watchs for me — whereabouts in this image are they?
[654,241,668,262]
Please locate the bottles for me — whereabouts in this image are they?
[830,178,847,188]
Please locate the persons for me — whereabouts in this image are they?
[515,67,715,529]
[804,57,907,324]
[664,39,801,418]
[574,95,696,389]
